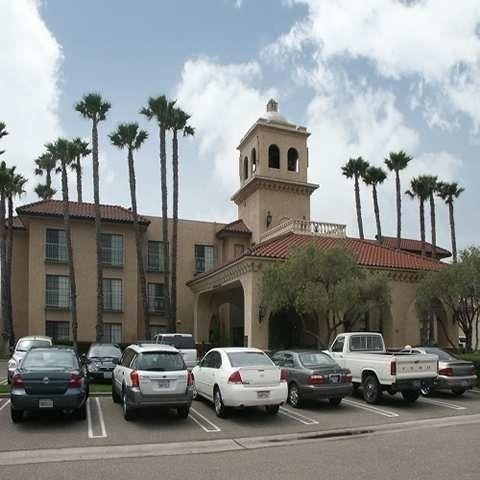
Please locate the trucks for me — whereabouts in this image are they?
[321,331,440,407]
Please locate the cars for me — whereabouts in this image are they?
[192,345,289,417]
[459,336,474,350]
[270,348,355,408]
[398,346,479,397]
[9,345,92,422]
[7,328,200,384]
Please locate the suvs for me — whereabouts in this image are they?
[111,340,192,418]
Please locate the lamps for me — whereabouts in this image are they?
[259,304,266,323]
[267,211,272,227]
